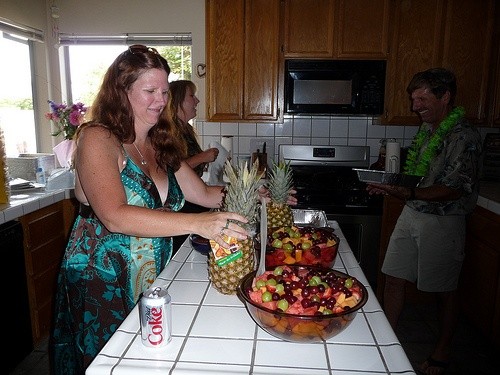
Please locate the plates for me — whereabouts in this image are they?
[6,153,57,191]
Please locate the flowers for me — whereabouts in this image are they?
[45,99,87,141]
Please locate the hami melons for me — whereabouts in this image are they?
[273,225,337,264]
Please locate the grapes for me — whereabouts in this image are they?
[256,268,356,333]
[266,227,332,258]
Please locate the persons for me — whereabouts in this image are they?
[366,66,483,375]
[47,44,297,375]
[169,80,219,256]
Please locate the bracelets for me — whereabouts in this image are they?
[406,188,415,201]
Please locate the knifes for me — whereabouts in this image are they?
[256,142,267,169]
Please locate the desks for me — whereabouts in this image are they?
[86,221,415,375]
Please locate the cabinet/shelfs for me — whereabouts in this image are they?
[20,198,81,345]
[205,0,284,123]
[384,0,500,126]
[284,0,393,59]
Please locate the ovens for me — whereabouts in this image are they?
[288,191,382,293]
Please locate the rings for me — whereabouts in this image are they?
[226,222,229,229]
[220,229,224,236]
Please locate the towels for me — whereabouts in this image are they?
[208,142,230,185]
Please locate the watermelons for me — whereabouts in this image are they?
[266,231,337,266]
[249,266,360,329]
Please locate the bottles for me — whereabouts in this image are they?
[35,168,46,184]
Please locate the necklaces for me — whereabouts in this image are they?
[133,142,147,165]
[403,106,466,177]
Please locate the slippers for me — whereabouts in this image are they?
[419,352,451,375]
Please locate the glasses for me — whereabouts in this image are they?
[128,45,158,58]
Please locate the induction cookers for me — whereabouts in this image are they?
[277,144,371,190]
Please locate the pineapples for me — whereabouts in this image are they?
[265,160,294,227]
[208,157,265,295]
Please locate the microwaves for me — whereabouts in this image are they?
[283,59,386,117]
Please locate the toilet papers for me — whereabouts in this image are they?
[40,155,55,171]
[386,143,401,172]
[221,137,233,182]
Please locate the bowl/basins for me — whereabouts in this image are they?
[238,264,369,344]
[254,225,341,270]
[189,235,211,256]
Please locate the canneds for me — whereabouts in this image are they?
[140,287,172,347]
